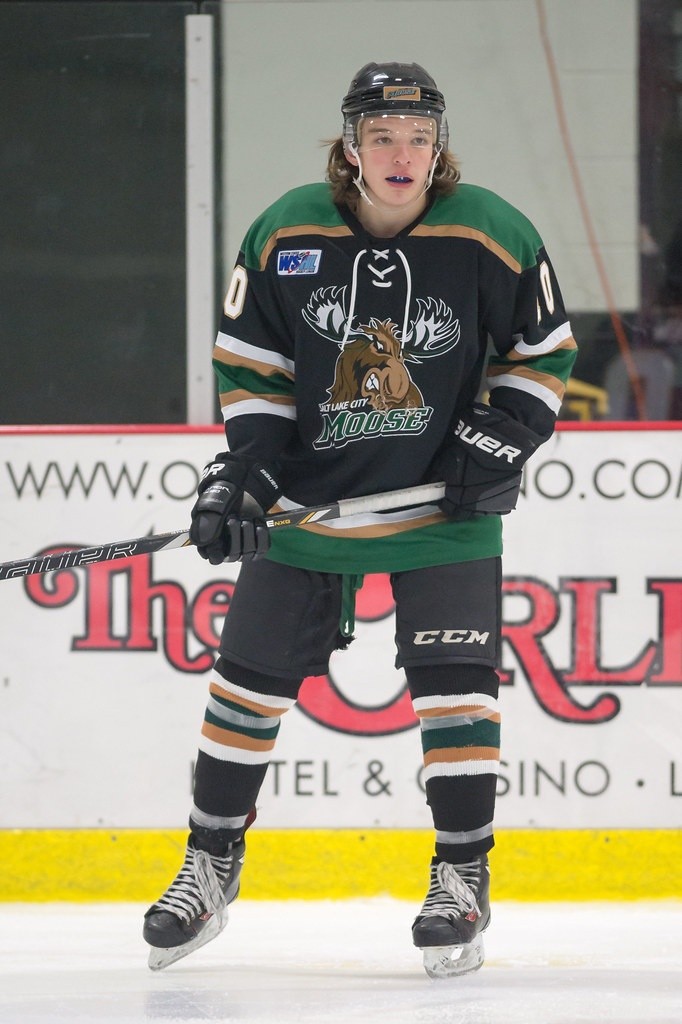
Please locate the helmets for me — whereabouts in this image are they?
[340,61,446,146]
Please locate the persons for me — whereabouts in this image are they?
[143,62,576,981]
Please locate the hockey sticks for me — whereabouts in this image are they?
[1,481,449,583]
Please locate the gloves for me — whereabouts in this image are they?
[428,401,548,522]
[190,451,284,565]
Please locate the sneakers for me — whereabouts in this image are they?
[411,853,491,979]
[143,806,257,971]
